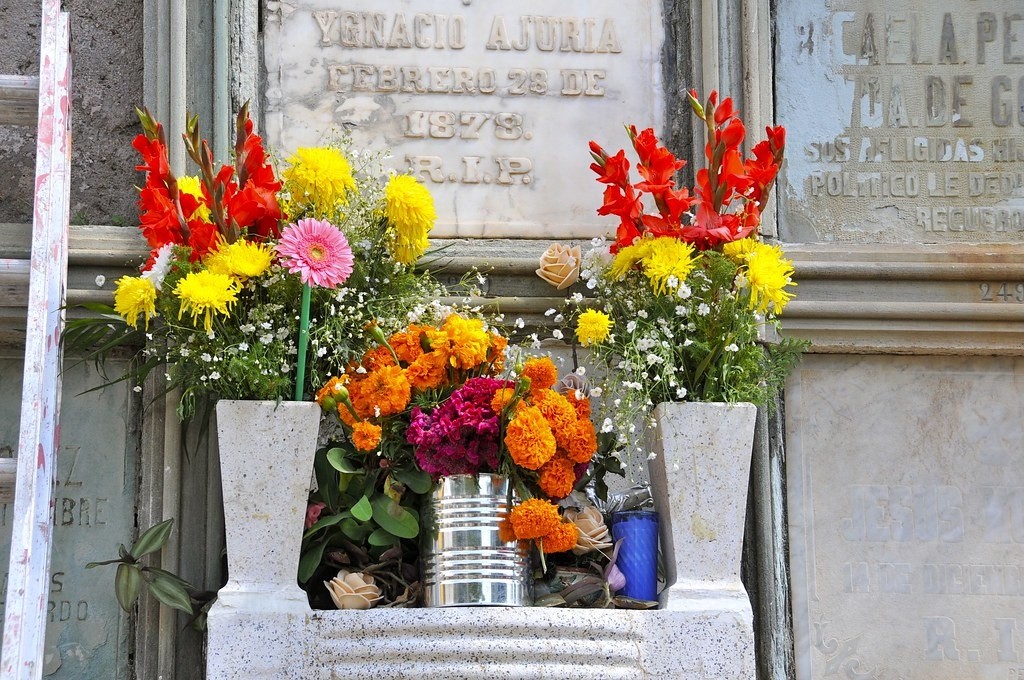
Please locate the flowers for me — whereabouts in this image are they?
[56,87,813,610]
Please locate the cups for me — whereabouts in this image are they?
[611,511,659,603]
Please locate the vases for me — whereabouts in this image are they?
[642,402,757,608]
[417,474,535,608]
[216,400,321,610]
[611,512,658,603]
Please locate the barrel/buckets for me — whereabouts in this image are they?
[423,474,530,607]
